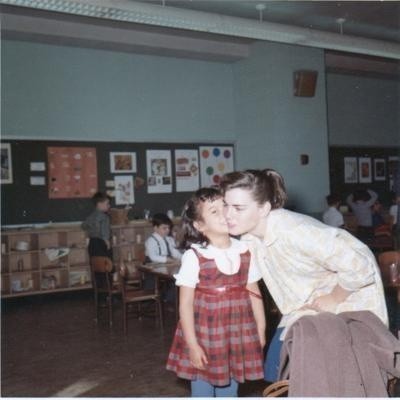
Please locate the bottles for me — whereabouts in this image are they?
[390,263,398,282]
[48,275,56,289]
[18,260,24,272]
[80,275,86,285]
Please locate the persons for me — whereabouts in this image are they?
[321,187,400,261]
[165,187,267,397]
[221,166,390,384]
[140,214,184,308]
[81,191,113,306]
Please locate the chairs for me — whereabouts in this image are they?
[90,255,163,338]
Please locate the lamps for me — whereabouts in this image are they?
[1,0,400,61]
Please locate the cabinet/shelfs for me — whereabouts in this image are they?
[0,221,153,299]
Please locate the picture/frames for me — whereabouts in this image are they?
[358,157,385,184]
[110,151,138,175]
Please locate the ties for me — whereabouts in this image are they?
[163,237,171,256]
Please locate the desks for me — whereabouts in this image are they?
[138,260,181,329]
